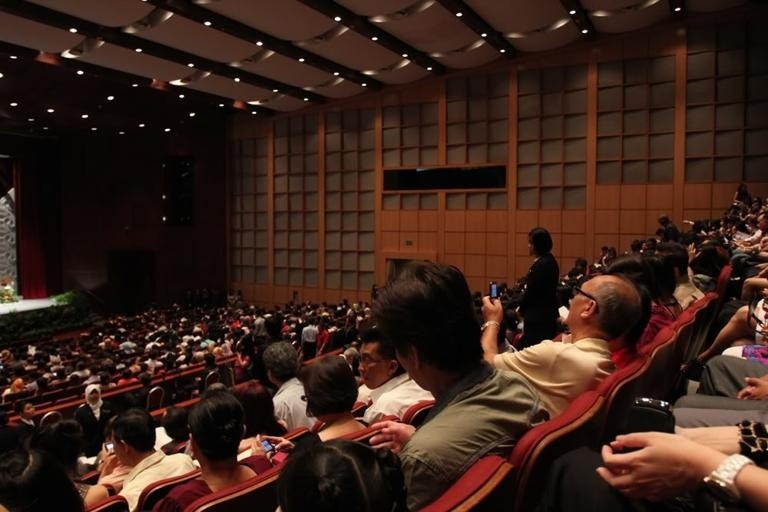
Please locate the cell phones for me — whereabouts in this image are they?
[261,440,276,454]
[105,441,114,451]
[489,281,498,300]
[621,397,675,451]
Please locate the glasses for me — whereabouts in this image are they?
[358,357,392,369]
[572,286,599,313]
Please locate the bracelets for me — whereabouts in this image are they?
[736,422,766,465]
[481,320,501,334]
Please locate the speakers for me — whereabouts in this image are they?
[164,155,195,225]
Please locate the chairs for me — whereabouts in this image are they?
[0,244,731,512]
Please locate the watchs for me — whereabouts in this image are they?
[700,451,755,508]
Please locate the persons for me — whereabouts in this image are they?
[559,184,768,364]
[474,279,524,340]
[517,229,560,348]
[477,272,644,423]
[0,425,90,511]
[370,257,552,510]
[251,357,365,475]
[541,421,767,511]
[664,406,768,426]
[277,434,403,512]
[670,353,768,412]
[4,291,375,475]
[157,390,273,512]
[111,408,196,511]
[31,420,110,507]
[358,334,435,422]
